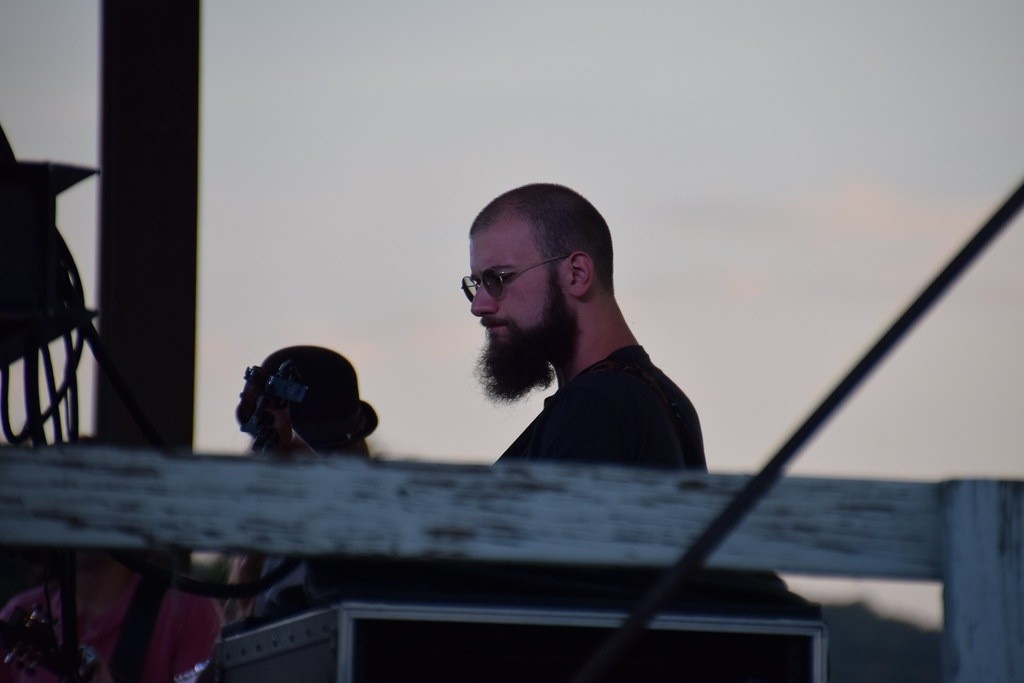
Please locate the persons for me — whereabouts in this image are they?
[461,184,711,471]
[235,345,378,458]
[0,558,225,681]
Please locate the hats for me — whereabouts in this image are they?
[237,345,377,450]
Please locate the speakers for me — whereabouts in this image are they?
[215,601,828,683]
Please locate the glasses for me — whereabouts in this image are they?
[461,253,570,302]
